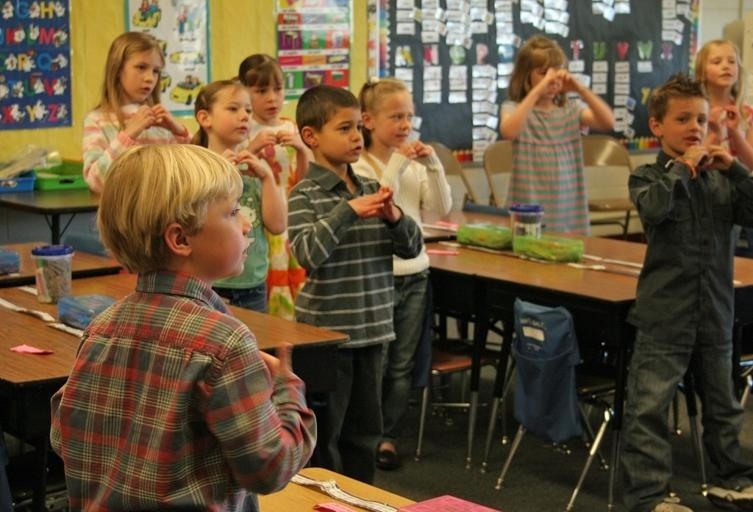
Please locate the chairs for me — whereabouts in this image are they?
[428,136,640,241]
[490,297,614,510]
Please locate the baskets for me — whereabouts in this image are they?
[0,162,88,193]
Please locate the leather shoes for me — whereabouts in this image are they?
[377,449,399,471]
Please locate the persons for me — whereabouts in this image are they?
[621,72,752,512]
[189,80,287,313]
[47,143,318,512]
[287,86,423,482]
[692,39,753,170]
[234,53,316,319]
[350,79,452,472]
[500,34,616,235]
[82,32,191,197]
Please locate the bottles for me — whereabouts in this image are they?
[453,149,472,162]
[617,136,658,152]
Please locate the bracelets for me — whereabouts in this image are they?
[675,157,697,179]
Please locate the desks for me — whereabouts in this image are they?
[419,208,751,512]
[0,241,121,288]
[0,188,99,245]
[413,285,509,462]
[255,465,417,512]
[0,276,351,469]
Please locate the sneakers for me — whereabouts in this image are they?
[708,483,753,507]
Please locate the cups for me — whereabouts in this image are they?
[27,243,75,302]
[506,204,543,245]
